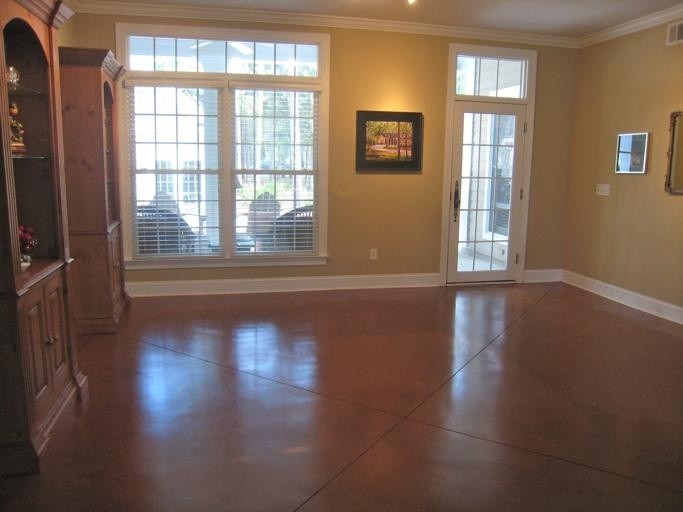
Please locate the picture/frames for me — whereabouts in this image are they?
[664,111,683,195]
[615,132,649,174]
[355,110,422,171]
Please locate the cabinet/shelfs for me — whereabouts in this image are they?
[58,46,131,334]
[0,0,90,473]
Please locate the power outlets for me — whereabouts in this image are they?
[369,249,375,262]
